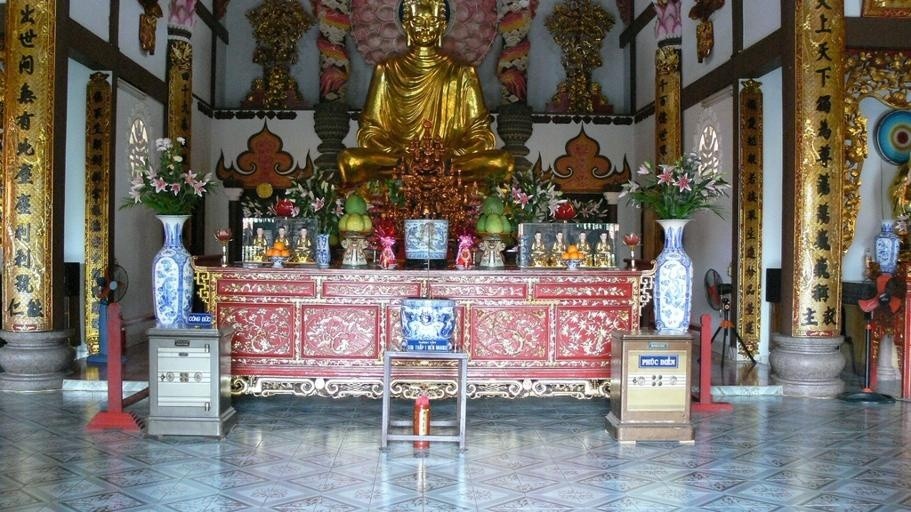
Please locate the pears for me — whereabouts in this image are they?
[338,192,372,232]
[476,195,512,233]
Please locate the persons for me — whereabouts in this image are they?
[337,0,516,192]
[251,224,271,260]
[596,231,614,261]
[293,226,315,261]
[551,229,568,261]
[530,230,552,262]
[577,231,594,260]
[273,226,291,249]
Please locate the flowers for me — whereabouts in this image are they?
[618,155,732,221]
[118,136,220,214]
[239,193,281,220]
[497,169,564,223]
[286,173,345,234]
[561,193,609,223]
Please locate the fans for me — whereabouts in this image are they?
[696,269,757,376]
[837,271,906,404]
[92,264,129,305]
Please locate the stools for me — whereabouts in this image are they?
[380,347,469,455]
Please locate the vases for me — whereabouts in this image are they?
[315,234,331,266]
[151,214,194,329]
[872,219,902,275]
[651,218,695,335]
